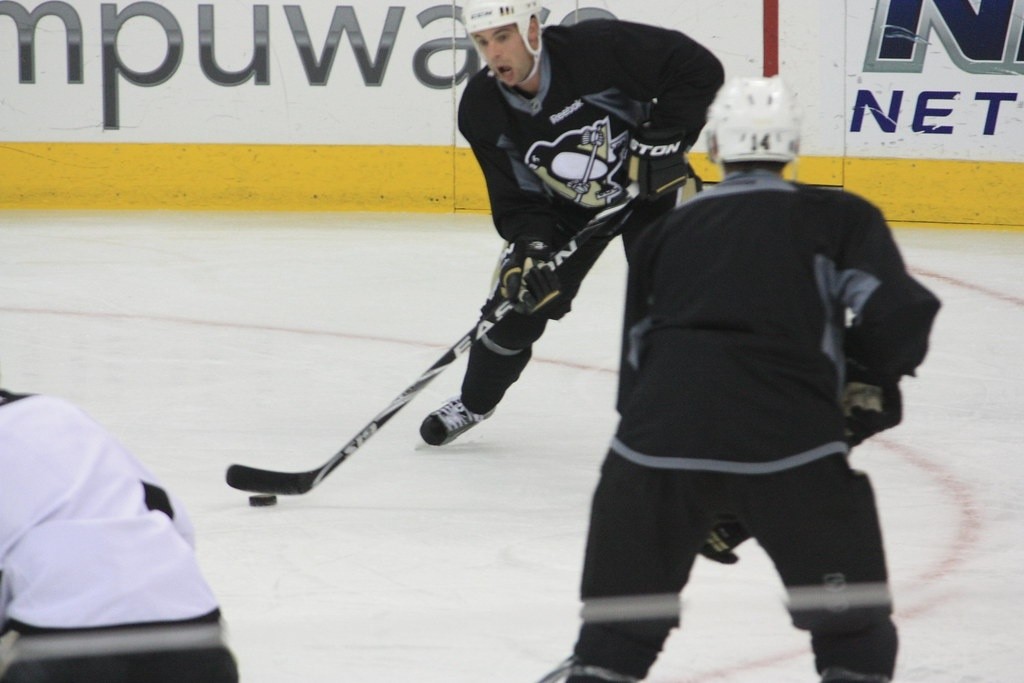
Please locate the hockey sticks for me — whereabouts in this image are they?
[536,392,883,682]
[224,195,625,498]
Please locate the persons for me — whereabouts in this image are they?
[566,78,942,683]
[419,0,725,446]
[0,389,240,683]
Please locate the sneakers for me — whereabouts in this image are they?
[416,397,496,451]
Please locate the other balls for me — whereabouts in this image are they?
[248,494,276,508]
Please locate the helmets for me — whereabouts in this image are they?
[460,0,542,56]
[705,74,801,164]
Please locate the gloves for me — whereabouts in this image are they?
[630,128,687,189]
[695,519,754,563]
[497,240,572,320]
[844,369,902,450]
